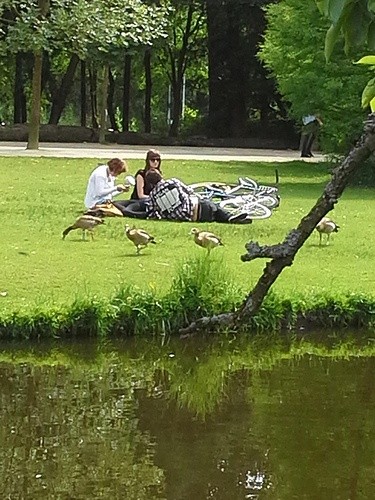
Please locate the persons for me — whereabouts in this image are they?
[83,157,129,217]
[143,169,252,225]
[301,111,323,157]
[130,149,164,200]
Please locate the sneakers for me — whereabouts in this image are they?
[301,152,313,157]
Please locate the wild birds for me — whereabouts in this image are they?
[62,215,105,240]
[315,217,340,246]
[126,224,156,255]
[188,228,225,256]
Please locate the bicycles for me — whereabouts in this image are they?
[191,170,280,219]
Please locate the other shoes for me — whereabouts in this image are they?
[228,212,248,224]
[236,218,252,224]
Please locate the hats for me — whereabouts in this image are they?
[148,151,160,159]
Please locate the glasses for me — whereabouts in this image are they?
[151,159,159,161]
